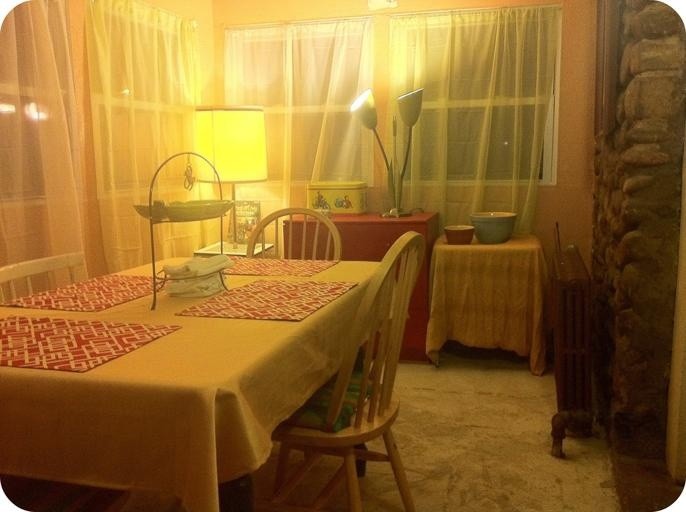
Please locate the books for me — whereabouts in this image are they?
[227,201,260,246]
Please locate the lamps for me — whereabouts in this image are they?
[189,104,271,251]
[350,86,433,217]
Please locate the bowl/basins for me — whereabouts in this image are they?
[133,204,166,221]
[469,210,518,244]
[165,201,236,222]
[444,225,474,245]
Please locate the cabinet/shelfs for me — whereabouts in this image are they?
[281,211,439,361]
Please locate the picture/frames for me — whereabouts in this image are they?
[223,197,259,243]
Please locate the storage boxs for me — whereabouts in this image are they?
[306,181,369,216]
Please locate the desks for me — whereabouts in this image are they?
[193,241,274,259]
[431,233,549,370]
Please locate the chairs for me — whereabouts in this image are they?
[0,249,95,306]
[264,232,429,507]
[247,205,347,263]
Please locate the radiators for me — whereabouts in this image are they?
[553,240,596,437]
